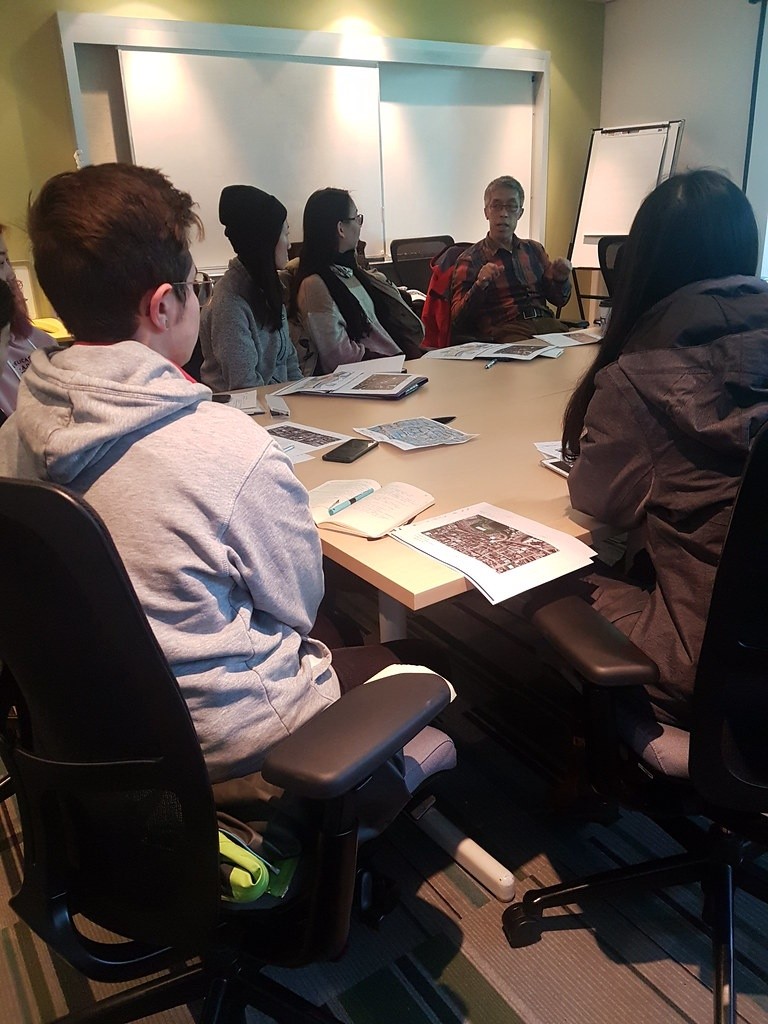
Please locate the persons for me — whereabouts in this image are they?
[198,182,306,396]
[0,225,60,419]
[0,163,470,820]
[295,186,427,377]
[450,174,574,345]
[559,168,768,734]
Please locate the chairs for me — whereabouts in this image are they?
[0,474,469,1024]
[500,413,768,1024]
[391,235,455,294]
[180,279,206,385]
[287,239,371,273]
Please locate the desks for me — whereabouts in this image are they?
[216,317,648,669]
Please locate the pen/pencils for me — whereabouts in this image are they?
[283,445,294,453]
[254,411,265,415]
[485,358,497,369]
[329,488,374,516]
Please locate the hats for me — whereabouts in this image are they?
[218,185,287,266]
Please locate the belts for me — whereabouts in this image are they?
[512,308,550,320]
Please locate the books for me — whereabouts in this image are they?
[305,478,435,540]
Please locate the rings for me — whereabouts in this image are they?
[482,277,486,281]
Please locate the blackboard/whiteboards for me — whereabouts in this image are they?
[567,118,686,270]
[114,45,535,272]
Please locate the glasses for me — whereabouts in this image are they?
[485,203,522,212]
[145,271,216,317]
[334,214,364,226]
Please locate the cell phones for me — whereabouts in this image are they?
[322,438,379,464]
[212,394,231,403]
